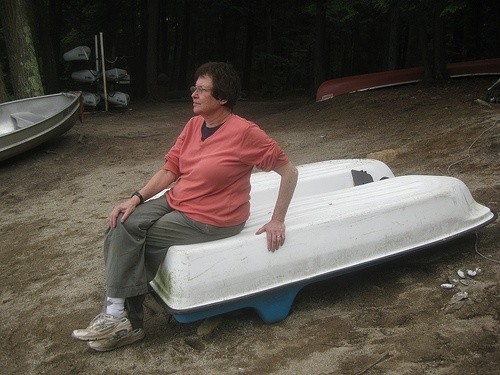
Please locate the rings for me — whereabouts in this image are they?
[277,235,281,238]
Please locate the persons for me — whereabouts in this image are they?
[71,62,299,354]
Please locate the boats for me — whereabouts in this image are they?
[0,90,84,165]
[315,57,500,102]
[117,156,495,327]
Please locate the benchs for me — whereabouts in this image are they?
[11,112,45,128]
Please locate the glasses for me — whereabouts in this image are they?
[190,86,214,94]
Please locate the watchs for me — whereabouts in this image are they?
[130,192,144,204]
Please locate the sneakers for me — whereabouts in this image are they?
[71,312,133,341]
[87,327,146,351]
